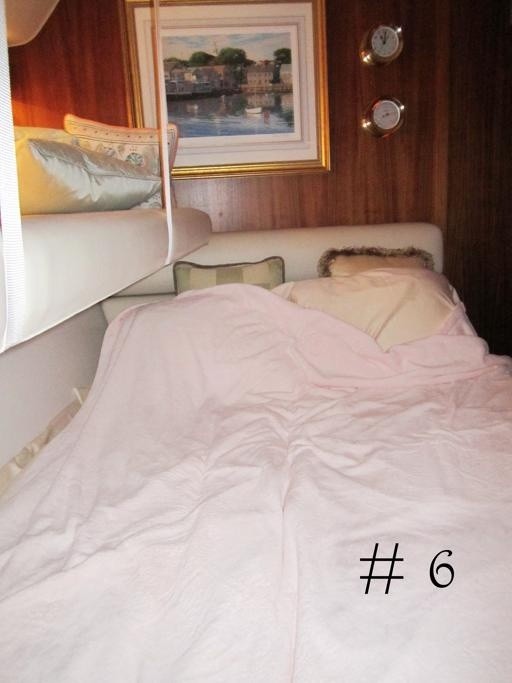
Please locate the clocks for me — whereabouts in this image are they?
[361,20,406,69]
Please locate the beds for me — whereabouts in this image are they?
[1,204,512,680]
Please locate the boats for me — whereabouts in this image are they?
[244,105,264,115]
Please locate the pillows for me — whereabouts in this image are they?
[63,112,179,209]
[173,255,286,296]
[17,135,164,215]
[316,244,435,278]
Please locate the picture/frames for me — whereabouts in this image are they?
[117,0,337,179]
